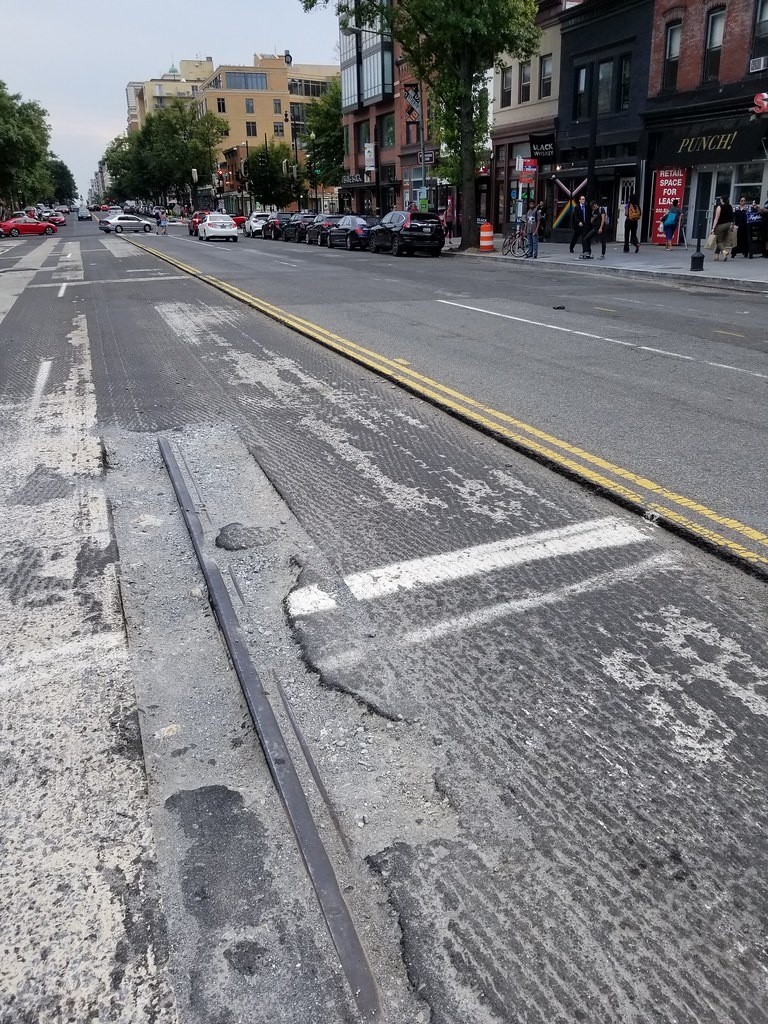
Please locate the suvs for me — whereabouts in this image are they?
[188,211,210,236]
[243,212,270,238]
[261,211,295,240]
[305,214,345,246]
[370,211,445,257]
[281,213,317,243]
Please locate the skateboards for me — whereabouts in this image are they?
[578,254,594,261]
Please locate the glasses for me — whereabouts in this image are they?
[741,200,746,202]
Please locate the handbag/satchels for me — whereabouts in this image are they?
[746,206,763,223]
[725,225,738,248]
[659,222,665,232]
[704,232,716,248]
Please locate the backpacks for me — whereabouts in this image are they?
[592,206,609,225]
[535,207,545,226]
[628,203,640,221]
[664,209,678,226]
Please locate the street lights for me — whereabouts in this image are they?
[283,111,301,212]
[340,25,426,189]
[241,140,253,216]
[310,130,318,215]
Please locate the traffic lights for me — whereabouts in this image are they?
[309,179,315,190]
[258,153,266,168]
[241,161,249,177]
[306,161,311,174]
[292,165,301,182]
[235,169,242,181]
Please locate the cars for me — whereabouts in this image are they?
[198,214,238,242]
[0,217,57,237]
[87,204,166,216]
[327,215,383,251]
[13,202,80,226]
[229,214,248,228]
[78,209,92,221]
[99,214,154,233]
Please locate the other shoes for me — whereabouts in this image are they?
[635,243,641,254]
[623,249,629,253]
[599,255,605,260]
[525,255,537,259]
[665,245,672,251]
[582,252,590,257]
[570,249,574,253]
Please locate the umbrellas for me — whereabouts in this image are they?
[24,205,36,210]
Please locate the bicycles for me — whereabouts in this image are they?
[502,219,530,258]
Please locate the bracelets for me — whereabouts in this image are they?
[712,228,715,229]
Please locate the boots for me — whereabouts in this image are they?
[712,254,719,261]
[722,250,729,262]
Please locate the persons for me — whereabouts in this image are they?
[569,195,591,253]
[184,208,188,218]
[180,206,183,219]
[155,208,168,235]
[392,204,397,211]
[38,209,42,221]
[538,201,546,242]
[524,200,542,259]
[444,205,455,244]
[408,202,418,212]
[623,194,641,254]
[755,191,768,258]
[731,196,750,258]
[581,200,607,261]
[347,207,354,215]
[29,208,34,218]
[660,200,681,250]
[711,194,733,262]
